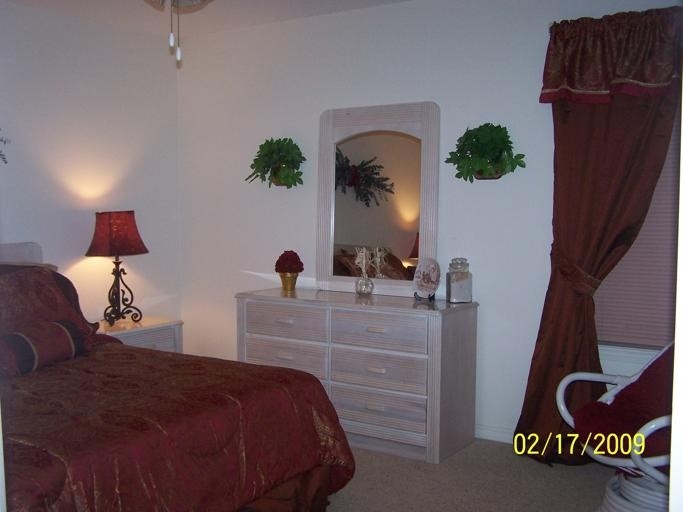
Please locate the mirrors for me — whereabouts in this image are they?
[315,101,440,299]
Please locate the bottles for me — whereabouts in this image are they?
[446,257,472,304]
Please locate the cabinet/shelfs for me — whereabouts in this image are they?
[235,287,480,464]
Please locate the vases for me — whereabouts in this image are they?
[279,272,298,295]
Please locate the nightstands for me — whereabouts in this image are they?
[90,316,185,354]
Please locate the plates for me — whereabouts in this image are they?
[412,258,441,298]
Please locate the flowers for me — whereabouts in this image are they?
[275,250,305,273]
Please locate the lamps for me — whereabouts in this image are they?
[84,210,149,325]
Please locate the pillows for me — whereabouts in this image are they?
[1,317,93,383]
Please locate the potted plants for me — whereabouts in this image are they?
[444,122,526,184]
[244,137,306,190]
[336,147,396,208]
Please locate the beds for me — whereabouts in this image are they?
[1,242,340,511]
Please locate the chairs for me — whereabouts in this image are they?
[555,338,673,512]
